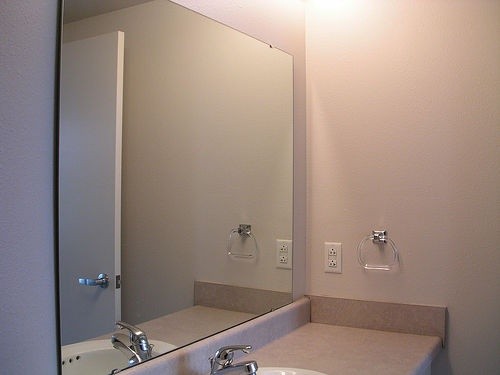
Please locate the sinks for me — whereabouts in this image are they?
[61,339,177,375]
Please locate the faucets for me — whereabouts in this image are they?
[111,320,152,367]
[208,344,258,375]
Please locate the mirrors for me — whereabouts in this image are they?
[52,1,297,375]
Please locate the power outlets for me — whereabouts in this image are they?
[324,242,342,273]
[275,239,293,271]
